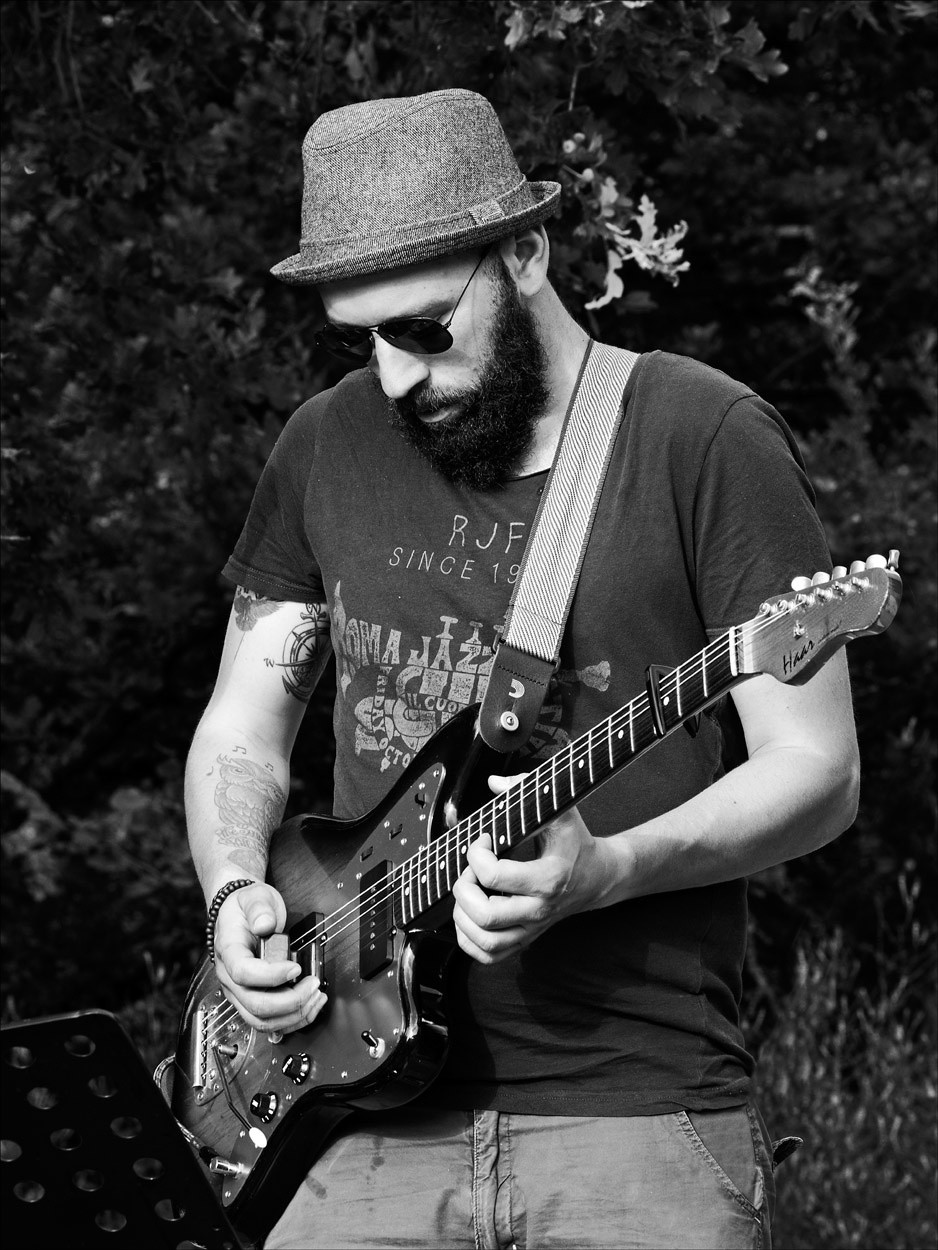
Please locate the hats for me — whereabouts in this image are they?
[270,89,561,287]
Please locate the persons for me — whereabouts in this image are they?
[184,88,861,1250]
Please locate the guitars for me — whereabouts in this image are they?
[165,549,905,1215]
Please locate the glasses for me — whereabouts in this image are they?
[313,246,490,365]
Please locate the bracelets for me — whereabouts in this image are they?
[206,879,256,965]
